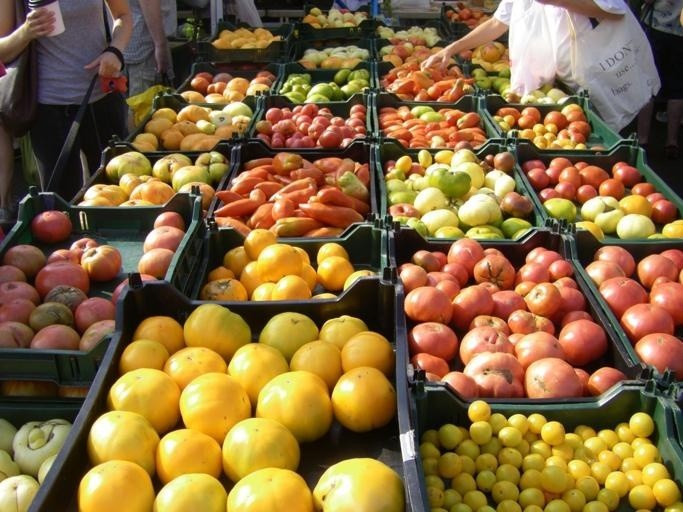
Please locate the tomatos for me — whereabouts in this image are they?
[525,157,676,222]
[398,236,629,400]
[0,210,184,354]
[584,244,683,387]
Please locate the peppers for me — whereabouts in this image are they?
[217,150,373,241]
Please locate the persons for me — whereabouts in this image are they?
[1,0,133,205]
[421,0,659,139]
[1,62,19,232]
[104,0,178,135]
[636,0,683,158]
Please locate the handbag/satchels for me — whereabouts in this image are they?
[0,1,39,137]
[127,72,176,128]
[567,4,661,134]
